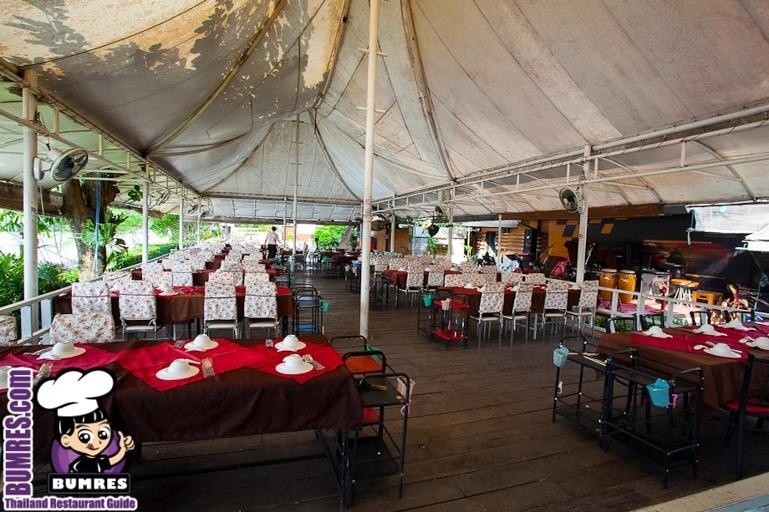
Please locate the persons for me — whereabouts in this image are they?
[263,227,281,266]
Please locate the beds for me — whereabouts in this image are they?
[0,334,366,511]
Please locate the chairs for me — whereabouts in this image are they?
[683,309,713,415]
[0,241,600,350]
[627,312,689,424]
[603,314,675,435]
[687,354,769,480]
[724,309,756,323]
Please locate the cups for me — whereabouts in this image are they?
[285,353,302,369]
[52,340,75,354]
[169,358,191,374]
[464,281,518,293]
[194,333,211,347]
[156,282,177,297]
[283,334,299,347]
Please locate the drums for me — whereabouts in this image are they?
[618,270,636,304]
[600,269,617,301]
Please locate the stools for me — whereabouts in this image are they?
[677,281,701,308]
[670,279,691,308]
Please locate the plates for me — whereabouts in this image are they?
[184,342,218,350]
[39,349,87,361]
[156,366,199,380]
[274,342,307,351]
[645,320,768,359]
[274,361,314,374]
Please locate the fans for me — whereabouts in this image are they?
[558,186,586,214]
[434,205,451,220]
[182,203,197,215]
[33,148,89,181]
[198,210,210,219]
[148,189,171,209]
